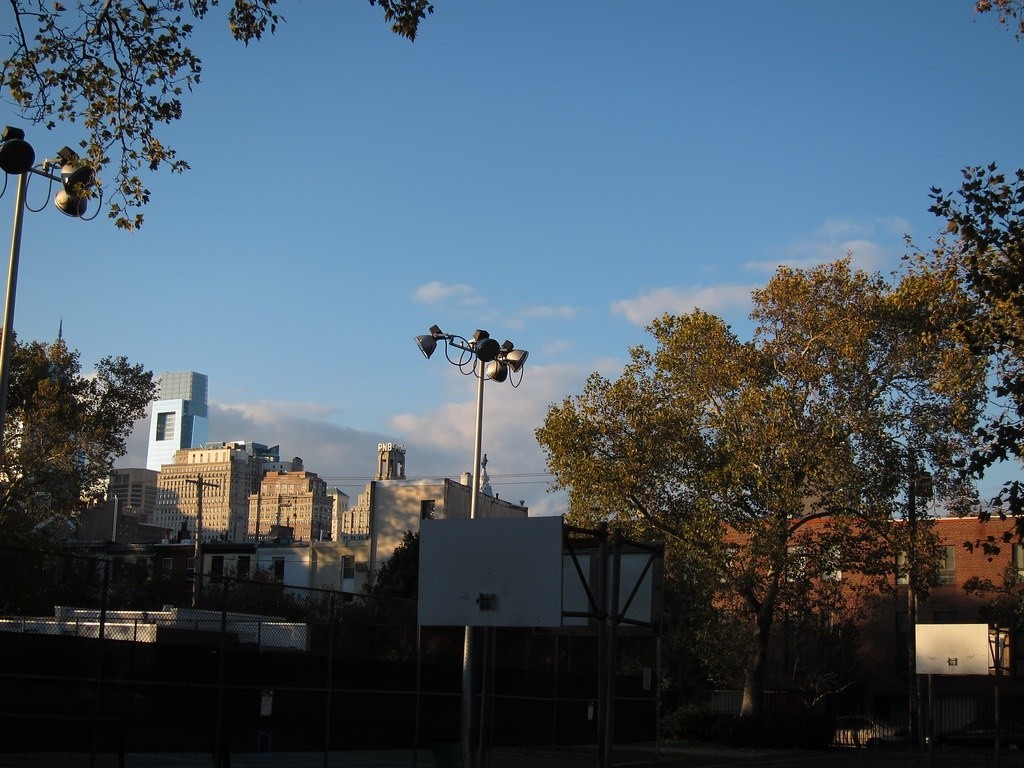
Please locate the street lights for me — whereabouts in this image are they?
[0,124,102,422]
[414,323,530,768]
